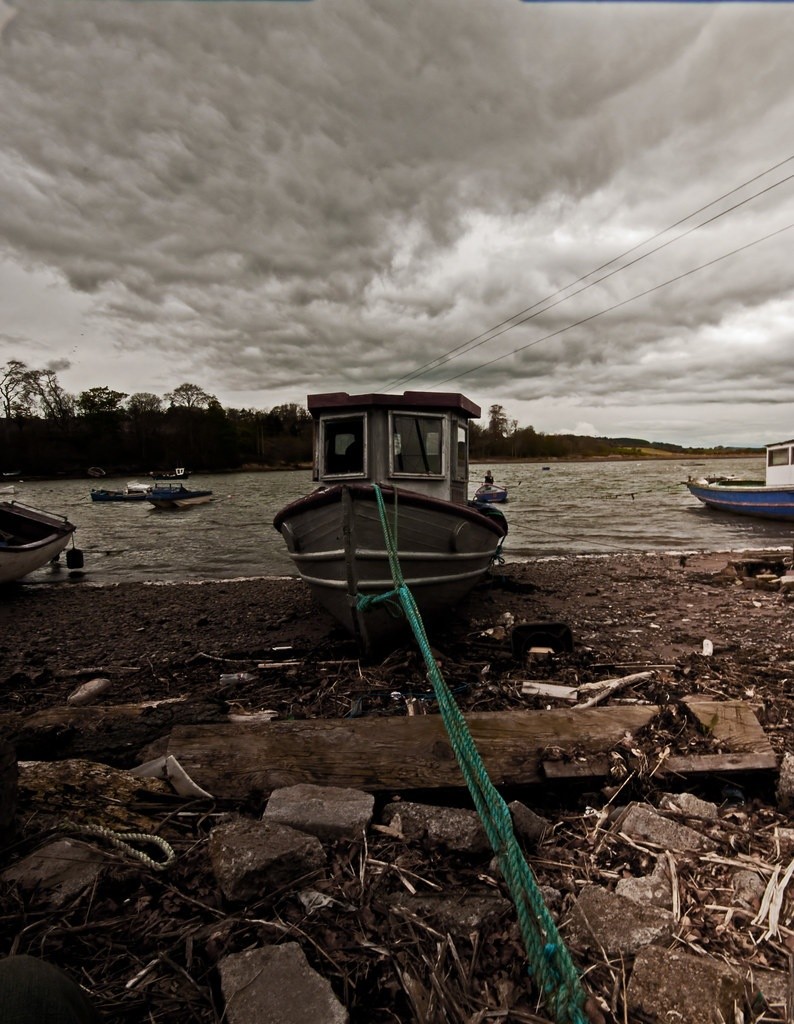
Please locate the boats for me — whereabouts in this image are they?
[686,439,794,522]
[91,467,213,511]
[272,391,508,640]
[0,500,77,585]
[475,483,508,503]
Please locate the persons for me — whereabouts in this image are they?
[485,470,493,490]
[344,433,364,473]
